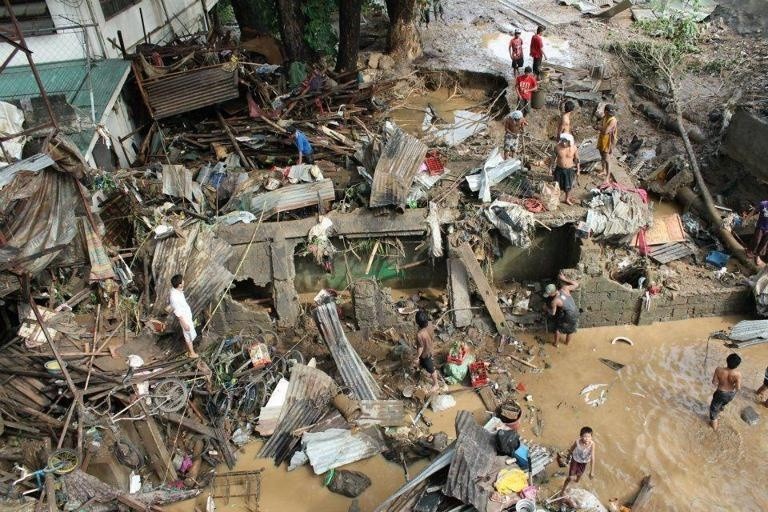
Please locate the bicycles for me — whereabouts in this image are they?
[191,323,304,424]
[7,448,81,500]
[82,378,189,469]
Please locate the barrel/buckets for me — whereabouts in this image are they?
[495,400,522,431]
[196,162,227,188]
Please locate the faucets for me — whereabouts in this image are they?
[516,498,535,511]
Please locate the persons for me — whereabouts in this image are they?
[515,66,538,117]
[547,133,580,206]
[542,272,579,348]
[509,27,522,77]
[503,110,525,161]
[559,425,596,498]
[530,25,547,80]
[411,310,440,393]
[752,264,768,321]
[167,274,206,360]
[709,353,742,432]
[741,200,768,267]
[592,103,616,186]
[755,365,768,408]
[286,127,316,171]
[556,100,574,145]
